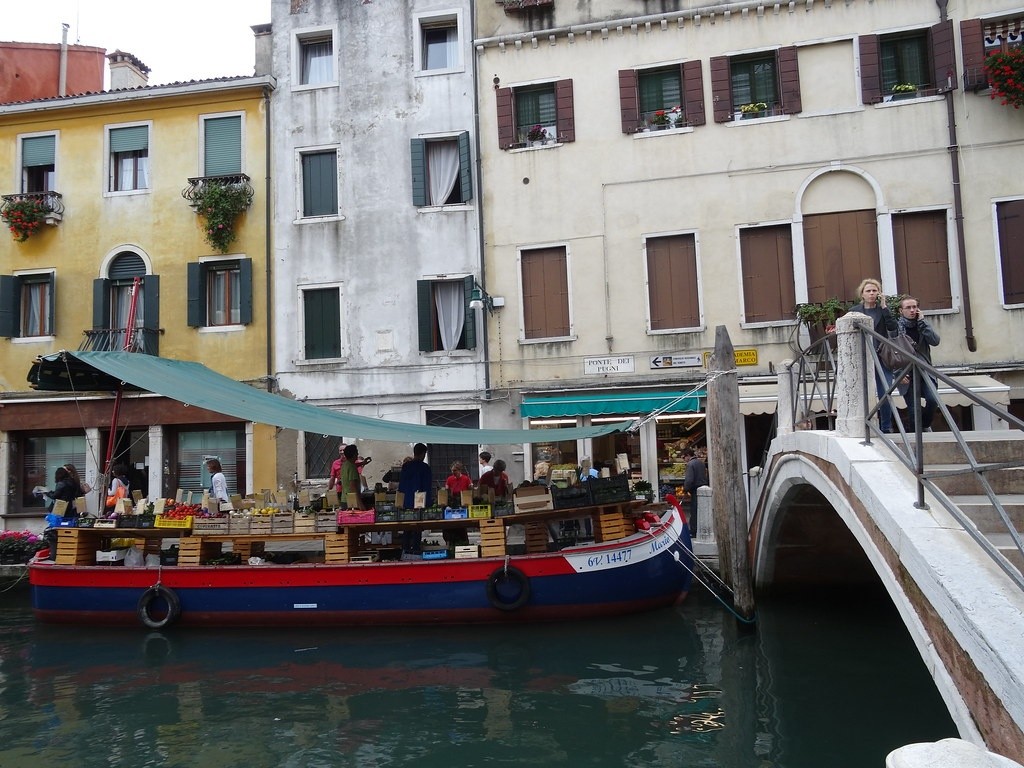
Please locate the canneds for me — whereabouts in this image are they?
[657,427,672,438]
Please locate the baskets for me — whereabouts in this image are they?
[337,503,492,523]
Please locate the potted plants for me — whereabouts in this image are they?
[796,295,900,330]
[632,480,656,502]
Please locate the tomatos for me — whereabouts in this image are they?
[162,505,199,519]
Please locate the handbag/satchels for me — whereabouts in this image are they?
[124,547,145,567]
[106,479,126,507]
[877,325,918,369]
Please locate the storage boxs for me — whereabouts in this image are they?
[96,474,630,562]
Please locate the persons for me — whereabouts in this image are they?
[328,444,372,510]
[578,456,598,481]
[681,448,707,538]
[108,466,130,513]
[207,459,228,510]
[848,280,899,433]
[398,442,432,555]
[478,452,508,495]
[44,464,81,518]
[890,295,940,432]
[446,462,473,498]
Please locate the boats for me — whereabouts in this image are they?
[30,492,694,631]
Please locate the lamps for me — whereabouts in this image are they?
[470,282,493,318]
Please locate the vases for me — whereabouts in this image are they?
[675,121,682,128]
[0,554,35,565]
[658,123,666,130]
[751,111,758,117]
[895,90,916,100]
[532,141,542,146]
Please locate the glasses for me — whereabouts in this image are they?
[903,305,917,309]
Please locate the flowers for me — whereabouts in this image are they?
[649,106,683,125]
[190,180,253,254]
[892,83,918,92]
[741,103,767,113]
[0,530,48,555]
[527,126,547,141]
[982,45,1024,110]
[2,199,54,244]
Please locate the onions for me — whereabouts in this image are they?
[229,508,251,519]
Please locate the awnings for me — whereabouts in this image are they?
[520,390,706,418]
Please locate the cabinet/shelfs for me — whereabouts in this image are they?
[658,438,682,464]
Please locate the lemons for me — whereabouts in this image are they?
[253,507,279,516]
